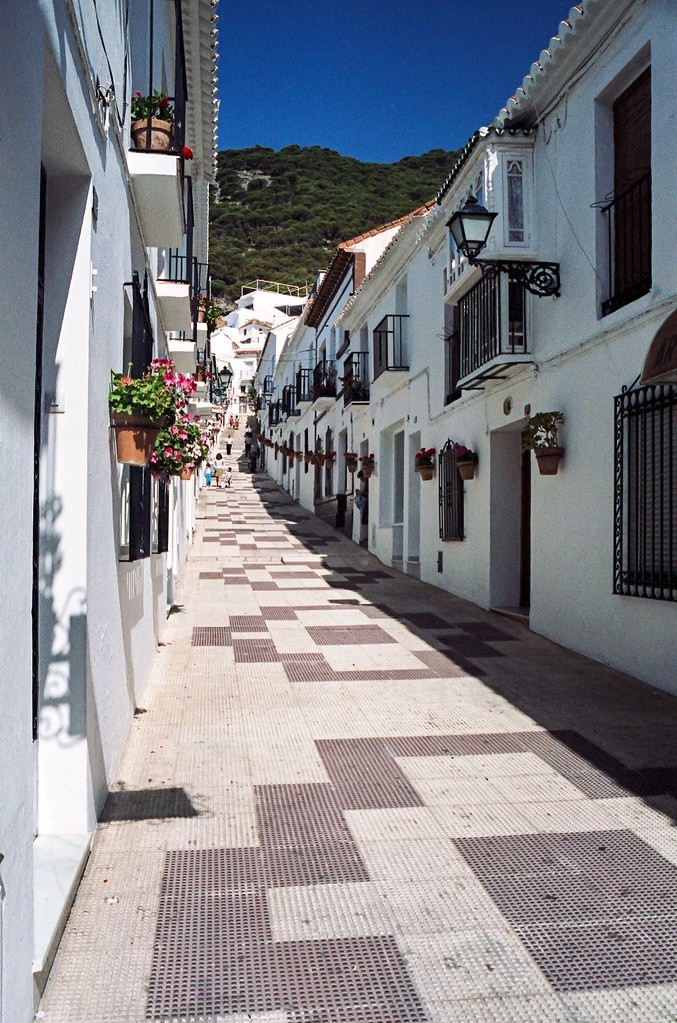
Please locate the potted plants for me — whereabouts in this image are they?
[519,411,565,475]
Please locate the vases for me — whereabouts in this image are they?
[193,306,206,323]
[457,461,475,480]
[363,466,372,478]
[420,465,434,480]
[326,460,334,468]
[347,461,357,472]
[113,412,164,467]
[180,468,192,480]
[129,118,174,150]
[258,436,324,466]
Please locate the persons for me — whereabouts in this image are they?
[213,453,226,487]
[244,434,253,458]
[225,434,233,455]
[234,415,239,430]
[228,415,233,429]
[223,467,232,488]
[203,463,212,487]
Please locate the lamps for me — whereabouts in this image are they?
[218,367,233,388]
[221,390,230,406]
[447,196,561,299]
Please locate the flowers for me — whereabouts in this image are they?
[416,448,436,464]
[110,359,196,422]
[455,443,476,461]
[359,454,374,465]
[132,90,175,126]
[152,415,210,481]
[324,452,336,459]
[339,377,362,389]
[343,453,358,460]
[190,294,220,323]
